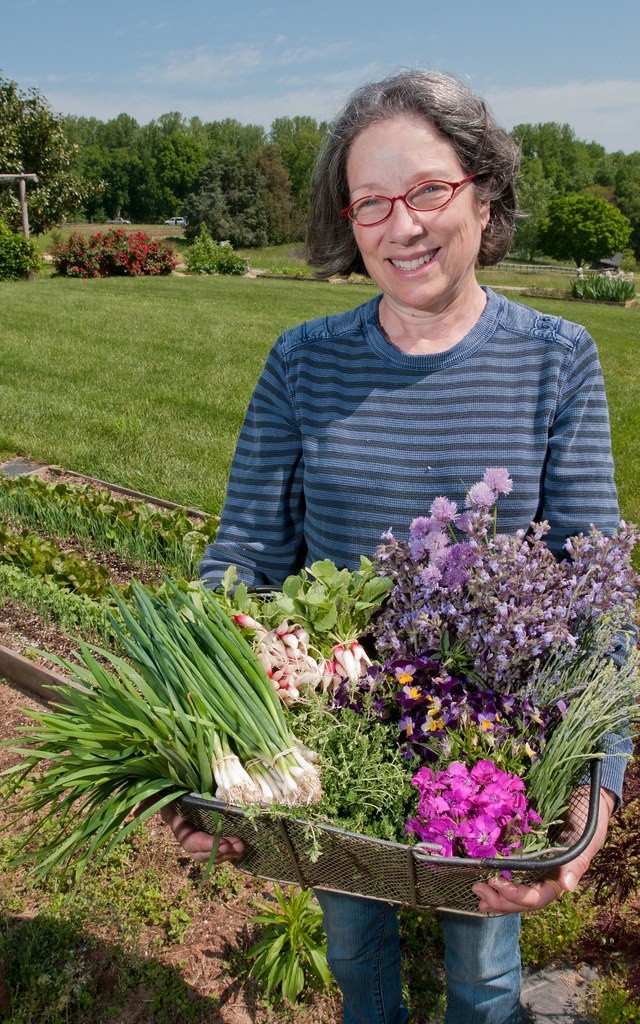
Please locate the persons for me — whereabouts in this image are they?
[162,71,633,1024]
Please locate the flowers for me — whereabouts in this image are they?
[329,466,640,887]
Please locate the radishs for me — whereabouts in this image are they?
[234,613,375,706]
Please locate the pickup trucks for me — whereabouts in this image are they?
[106,217,132,224]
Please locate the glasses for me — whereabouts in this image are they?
[339,169,493,228]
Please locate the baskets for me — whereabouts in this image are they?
[158,671,603,919]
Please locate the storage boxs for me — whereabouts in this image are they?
[161,727,606,918]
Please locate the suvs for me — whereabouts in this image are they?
[164,217,186,225]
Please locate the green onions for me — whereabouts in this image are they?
[99,570,330,811]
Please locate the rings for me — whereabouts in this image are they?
[545,878,562,900]
